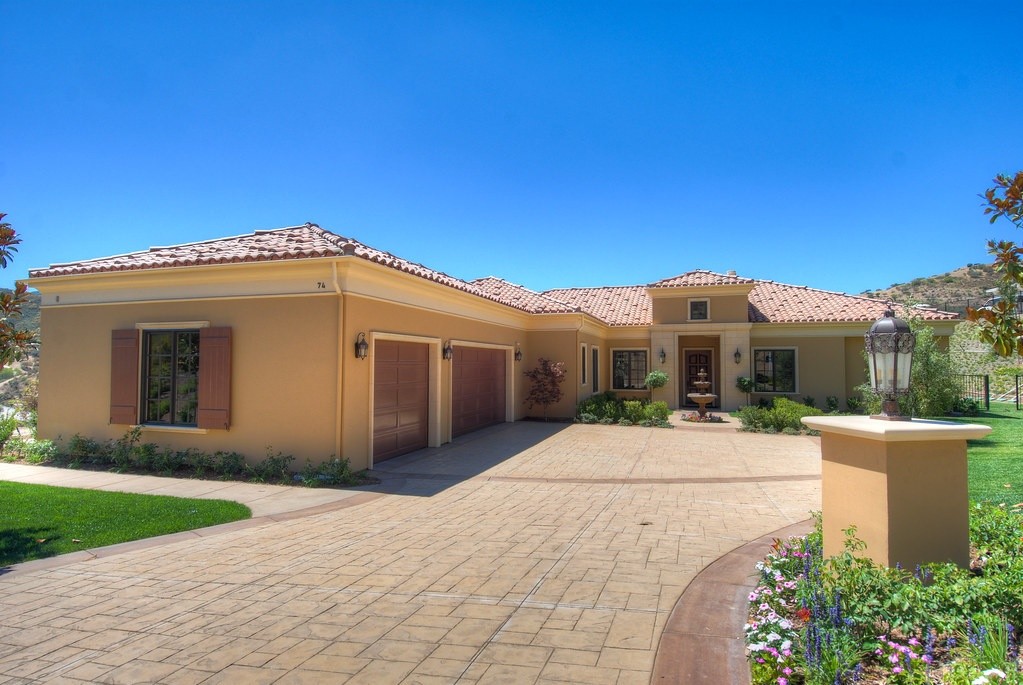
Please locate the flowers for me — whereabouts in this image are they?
[681,411,724,423]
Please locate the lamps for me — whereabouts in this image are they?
[443,340,454,362]
[734,348,741,365]
[515,347,522,362]
[865,297,918,422]
[354,332,368,361]
[659,348,666,366]
[943,378,964,417]
